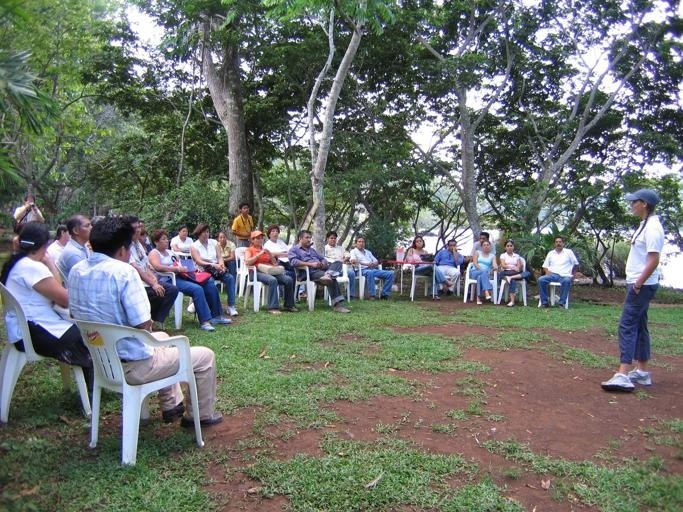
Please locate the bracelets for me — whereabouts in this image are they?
[634,284,642,290]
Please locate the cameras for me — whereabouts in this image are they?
[29,202,34,207]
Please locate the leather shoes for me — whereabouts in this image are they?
[476,301,482,305]
[485,295,491,300]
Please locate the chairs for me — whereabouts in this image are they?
[497,257,527,306]
[188,247,437,313]
[436,265,461,296]
[463,262,498,304]
[68,317,206,465]
[155,271,183,331]
[0,285,92,424]
[538,275,572,310]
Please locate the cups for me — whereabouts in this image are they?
[396,246,405,261]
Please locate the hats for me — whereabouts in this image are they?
[624,188,660,207]
[250,231,265,241]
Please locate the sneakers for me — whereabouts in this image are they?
[209,318,231,324]
[226,305,238,316]
[627,365,651,385]
[267,308,281,315]
[180,411,222,427]
[186,302,196,314]
[283,306,298,312]
[505,301,515,307]
[198,323,215,331]
[538,304,548,308]
[333,306,351,313]
[161,401,185,424]
[600,373,635,391]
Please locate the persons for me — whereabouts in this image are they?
[67,217,223,427]
[2,194,393,395]
[601,189,664,393]
[403,232,579,307]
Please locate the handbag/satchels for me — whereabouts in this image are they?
[172,261,212,285]
[202,265,229,280]
[13,223,20,233]
[257,262,284,275]
